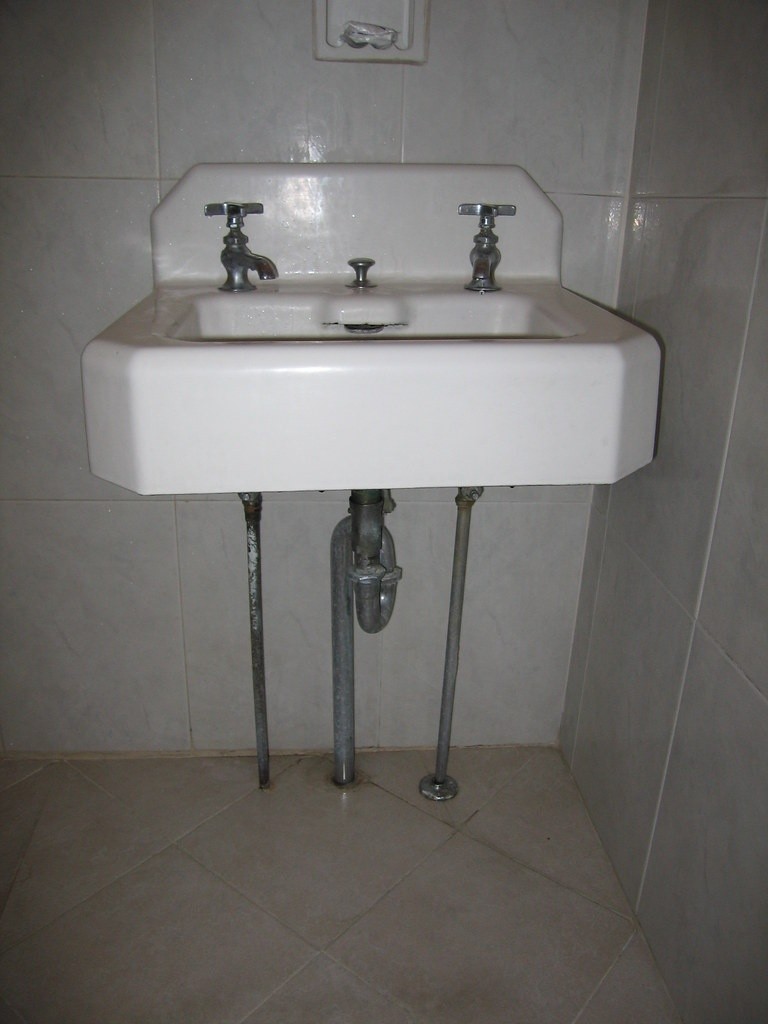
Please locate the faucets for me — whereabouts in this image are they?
[457,204,516,294]
[205,203,280,293]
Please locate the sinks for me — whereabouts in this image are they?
[82,163,662,496]
